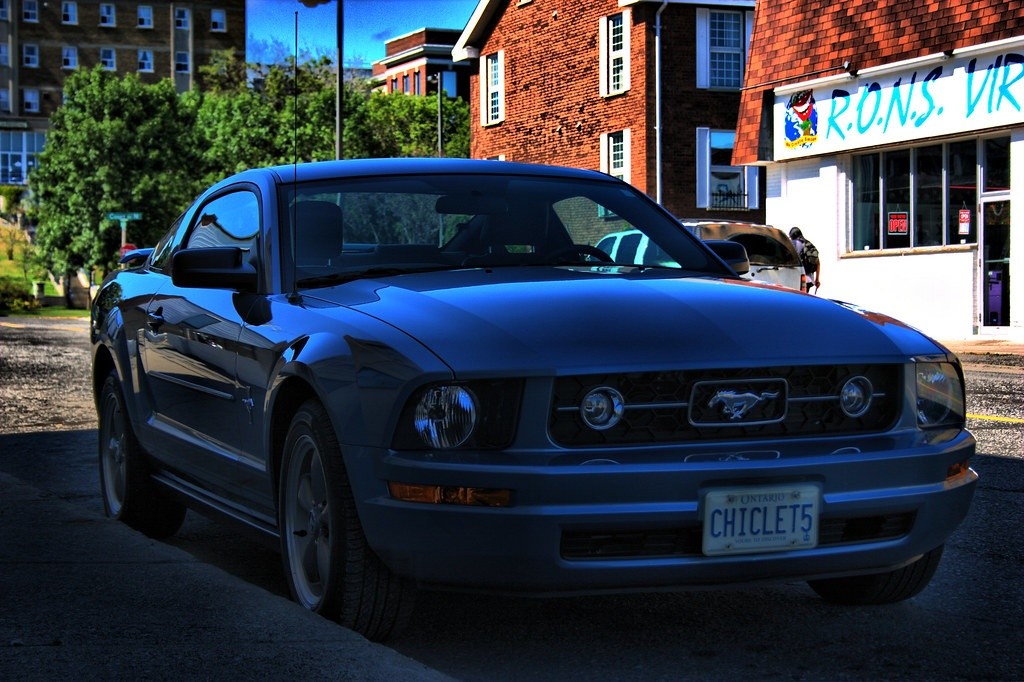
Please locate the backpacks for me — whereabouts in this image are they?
[795,237,819,275]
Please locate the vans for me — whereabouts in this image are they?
[586,222,806,296]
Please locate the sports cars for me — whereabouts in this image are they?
[91,159,978,644]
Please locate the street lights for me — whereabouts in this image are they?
[428,72,442,160]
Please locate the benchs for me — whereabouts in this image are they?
[336,243,447,273]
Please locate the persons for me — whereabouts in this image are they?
[790,227,820,293]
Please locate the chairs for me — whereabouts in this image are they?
[287,198,352,275]
[476,193,553,266]
